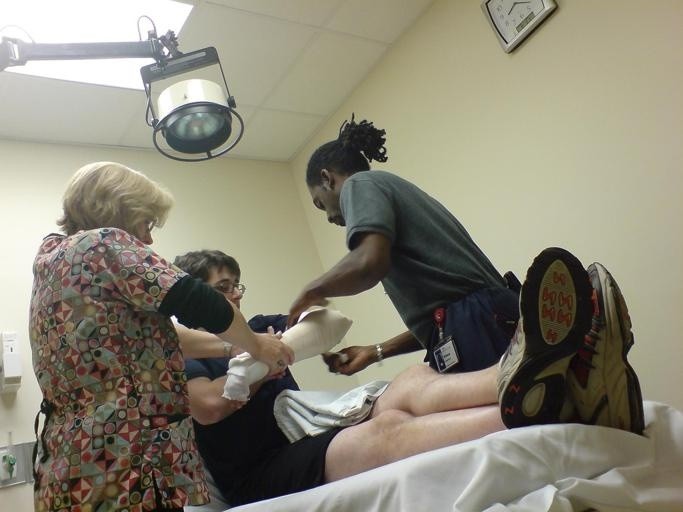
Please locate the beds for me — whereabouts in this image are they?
[182,400,682,511]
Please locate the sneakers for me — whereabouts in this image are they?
[497,248,645,435]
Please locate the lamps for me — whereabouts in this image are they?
[0,30,244,161]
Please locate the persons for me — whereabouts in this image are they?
[159,246,648,507]
[26,160,296,512]
[278,110,525,375]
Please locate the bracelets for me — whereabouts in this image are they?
[375,344,384,363]
[222,341,233,357]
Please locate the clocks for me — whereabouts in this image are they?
[480,0,557,54]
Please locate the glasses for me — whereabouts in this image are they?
[219,282,245,293]
